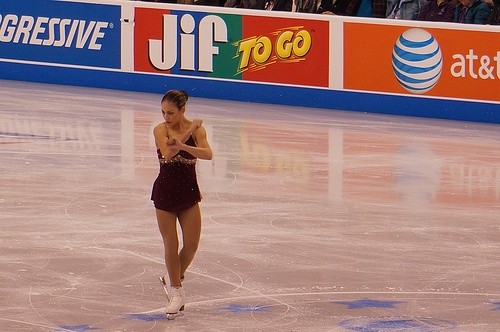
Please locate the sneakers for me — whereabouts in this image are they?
[159,271,185,302]
[165,287,185,321]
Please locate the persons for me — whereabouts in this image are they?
[191,0,500,26]
[150,89,213,320]
